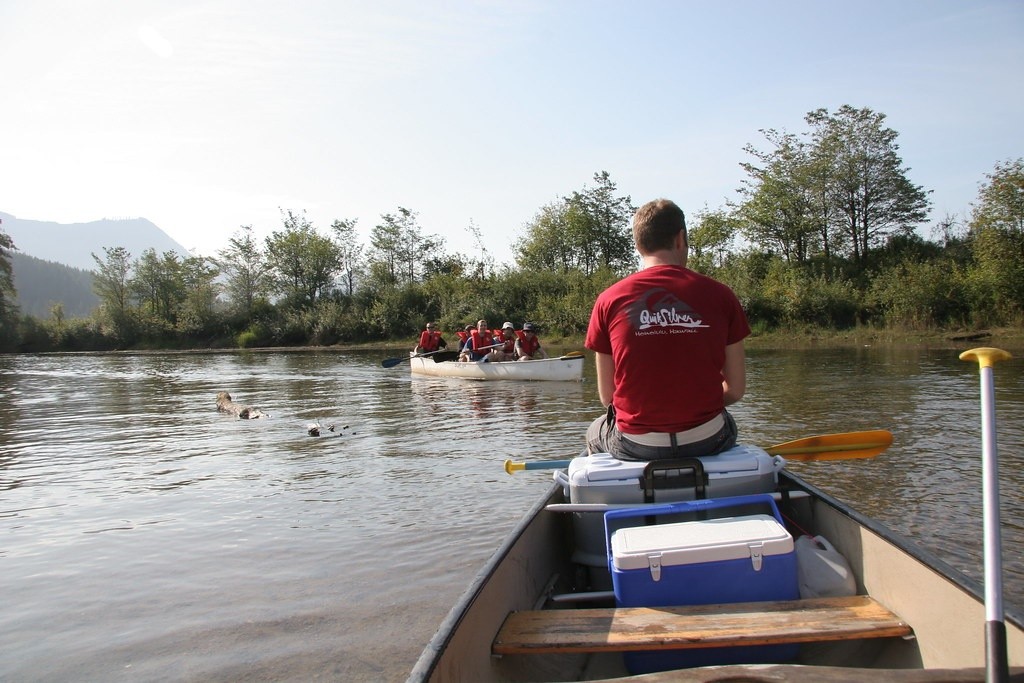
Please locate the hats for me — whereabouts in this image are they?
[502,322,515,333]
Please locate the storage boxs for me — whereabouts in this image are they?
[552,445,787,601]
[604,494,801,608]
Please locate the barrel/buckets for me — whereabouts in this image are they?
[795,534,857,599]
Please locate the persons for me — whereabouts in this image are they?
[585,197,753,455]
[418,322,449,360]
[456,320,549,362]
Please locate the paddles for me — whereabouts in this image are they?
[503,429,894,476]
[432,342,503,363]
[381,349,443,368]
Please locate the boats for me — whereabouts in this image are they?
[407,345,584,383]
[401,439,1023,683]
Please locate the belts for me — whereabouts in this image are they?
[615,413,726,447]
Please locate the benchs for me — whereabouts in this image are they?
[492,595,912,660]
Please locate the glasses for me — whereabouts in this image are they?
[427,327,435,329]
[523,329,536,334]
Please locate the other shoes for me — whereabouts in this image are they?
[483,357,489,363]
